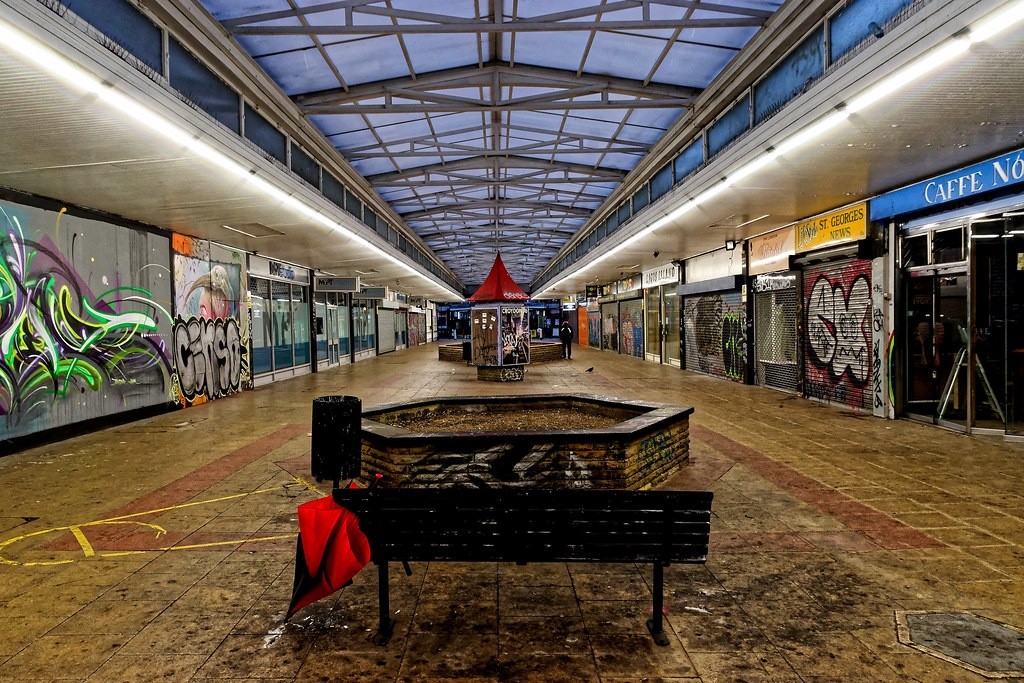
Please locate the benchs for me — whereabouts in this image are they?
[332,487,713,647]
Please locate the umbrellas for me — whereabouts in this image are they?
[282,474,399,627]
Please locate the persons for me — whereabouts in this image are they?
[560,321,574,359]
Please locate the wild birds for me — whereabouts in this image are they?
[585,366,594,373]
